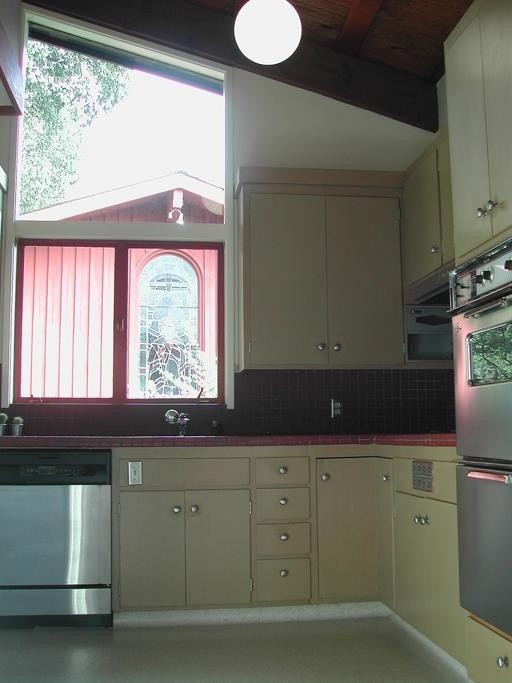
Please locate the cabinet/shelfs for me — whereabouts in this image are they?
[237,183,403,370]
[112,446,313,628]
[313,445,392,626]
[400,129,456,290]
[443,0,511,269]
[393,444,510,683]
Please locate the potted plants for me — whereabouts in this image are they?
[0,413,25,436]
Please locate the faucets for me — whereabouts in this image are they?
[165,410,190,435]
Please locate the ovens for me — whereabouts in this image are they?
[446,234,512,636]
